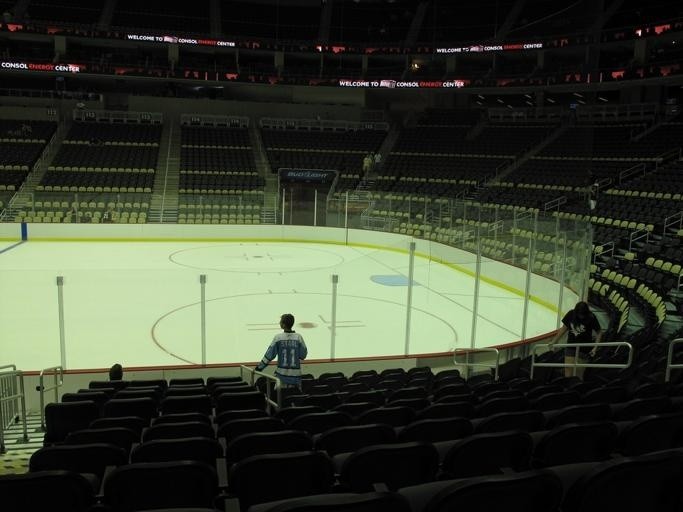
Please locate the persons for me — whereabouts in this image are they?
[361,155,372,176]
[253,312,308,394]
[588,186,598,210]
[546,301,603,382]
[108,362,123,381]
[373,151,381,165]
